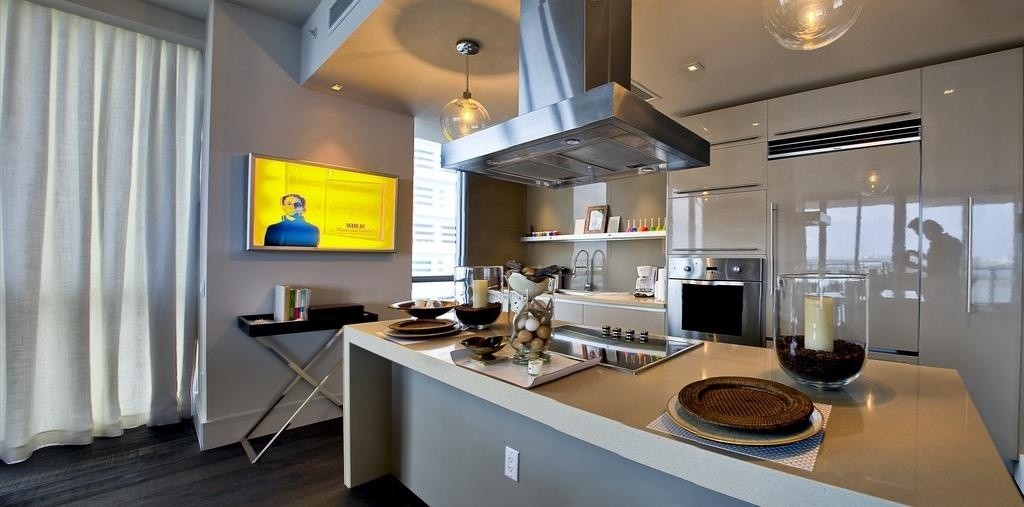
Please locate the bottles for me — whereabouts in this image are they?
[625,217,667,232]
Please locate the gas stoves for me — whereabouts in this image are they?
[548,321,704,371]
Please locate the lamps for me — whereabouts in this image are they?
[757,1,863,52]
[439,39,491,141]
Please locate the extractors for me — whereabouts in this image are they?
[439,0,710,190]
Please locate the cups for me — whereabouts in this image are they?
[774,272,869,392]
[526,359,543,375]
[532,230,558,236]
[503,272,554,365]
[454,266,504,328]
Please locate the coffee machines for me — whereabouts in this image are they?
[631,264,655,298]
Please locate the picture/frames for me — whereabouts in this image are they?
[584,204,608,233]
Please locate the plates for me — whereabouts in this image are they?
[665,377,825,446]
[383,318,459,337]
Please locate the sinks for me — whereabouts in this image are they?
[555,288,628,297]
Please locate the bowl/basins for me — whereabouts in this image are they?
[655,265,667,302]
[391,299,456,317]
[461,333,509,360]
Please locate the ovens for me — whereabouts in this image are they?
[665,254,763,343]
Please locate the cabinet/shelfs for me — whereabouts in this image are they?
[235,302,381,471]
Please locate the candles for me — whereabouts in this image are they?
[803,292,835,354]
[473,279,488,309]
[626,217,666,228]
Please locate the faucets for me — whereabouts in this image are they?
[570,249,590,286]
[592,250,606,286]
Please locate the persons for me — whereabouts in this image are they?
[264,193,320,247]
